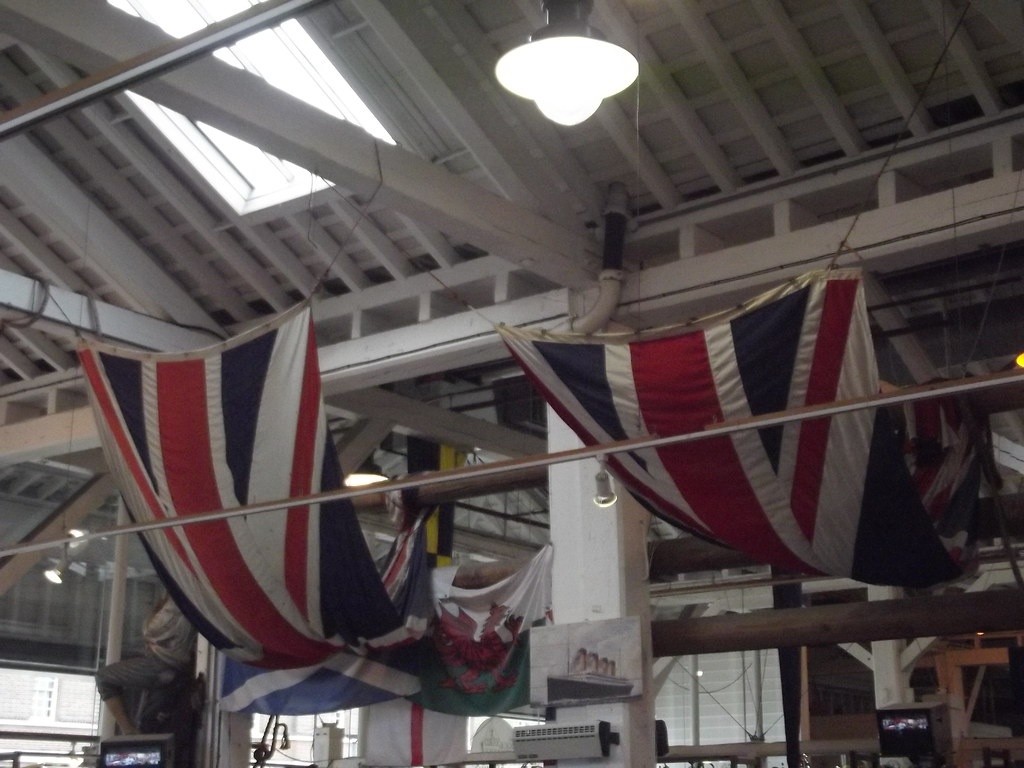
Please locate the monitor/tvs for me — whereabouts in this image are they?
[878,701,952,758]
[99,733,177,768]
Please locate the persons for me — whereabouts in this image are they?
[95,598,194,768]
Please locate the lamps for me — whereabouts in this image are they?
[492,0,640,126]
[344,448,389,488]
[593,454,618,508]
[42,561,64,584]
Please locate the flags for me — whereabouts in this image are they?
[78,303,439,665]
[503,277,979,584]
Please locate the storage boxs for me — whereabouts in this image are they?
[313,722,345,768]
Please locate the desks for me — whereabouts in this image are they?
[422,751,516,768]
